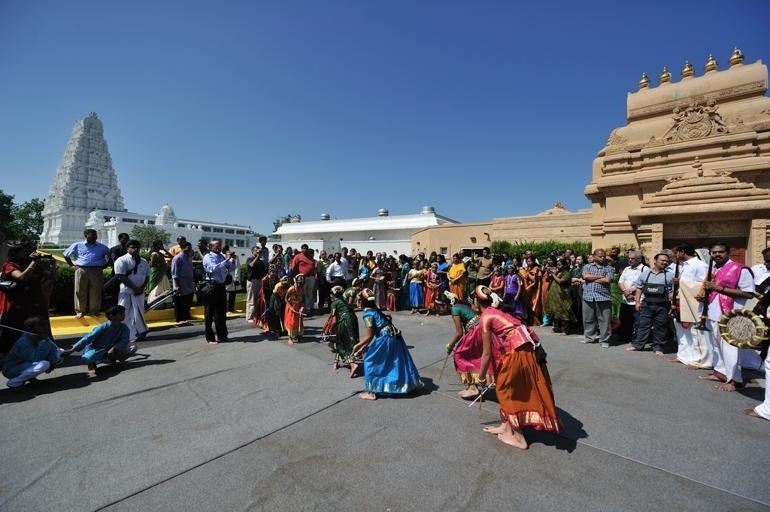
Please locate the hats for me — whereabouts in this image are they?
[268,260,516,304]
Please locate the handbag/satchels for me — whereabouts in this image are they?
[0,279,19,291]
[194,280,215,297]
[642,283,669,302]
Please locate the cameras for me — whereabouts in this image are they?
[259,248,266,255]
[547,268,552,272]
[230,251,236,258]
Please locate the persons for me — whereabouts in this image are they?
[468,284,565,451]
[147,236,770,420]
[1,229,151,390]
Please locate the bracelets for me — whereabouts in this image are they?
[477,377,487,383]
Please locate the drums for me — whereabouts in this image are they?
[718,309,770,350]
